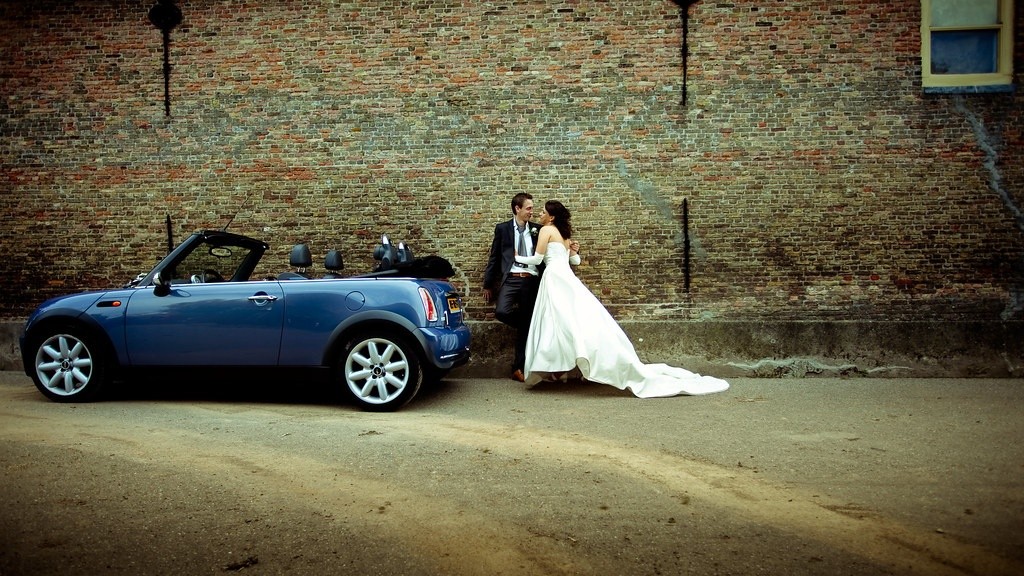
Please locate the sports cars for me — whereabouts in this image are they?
[18,228,472,411]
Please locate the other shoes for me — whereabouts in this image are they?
[542,372,568,383]
[511,369,524,382]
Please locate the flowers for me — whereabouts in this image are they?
[531,227,538,236]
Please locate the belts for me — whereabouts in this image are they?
[509,273,532,277]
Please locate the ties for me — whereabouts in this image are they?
[514,222,527,268]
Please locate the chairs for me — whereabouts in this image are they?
[322,249,344,280]
[373,234,416,271]
[278,244,315,280]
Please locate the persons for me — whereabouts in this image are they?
[515,201,730,398]
[481,193,580,382]
